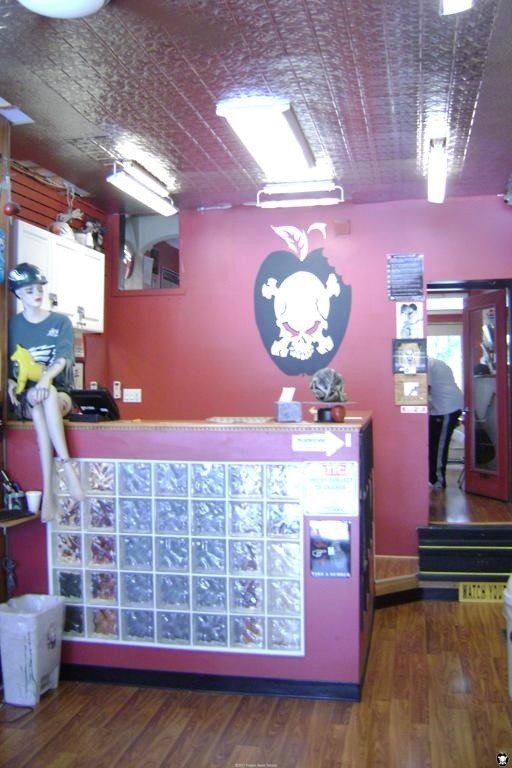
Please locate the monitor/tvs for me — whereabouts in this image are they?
[70,389,120,419]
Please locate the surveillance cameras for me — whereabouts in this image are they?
[503,193,512,207]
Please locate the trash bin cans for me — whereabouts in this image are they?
[0,594,66,706]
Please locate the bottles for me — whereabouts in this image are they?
[317,405,346,422]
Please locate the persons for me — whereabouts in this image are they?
[7,262,87,524]
[427,357,465,488]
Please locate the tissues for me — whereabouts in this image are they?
[274,386,303,422]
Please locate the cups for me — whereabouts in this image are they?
[3,490,42,515]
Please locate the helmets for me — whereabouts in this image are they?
[8,262,48,292]
